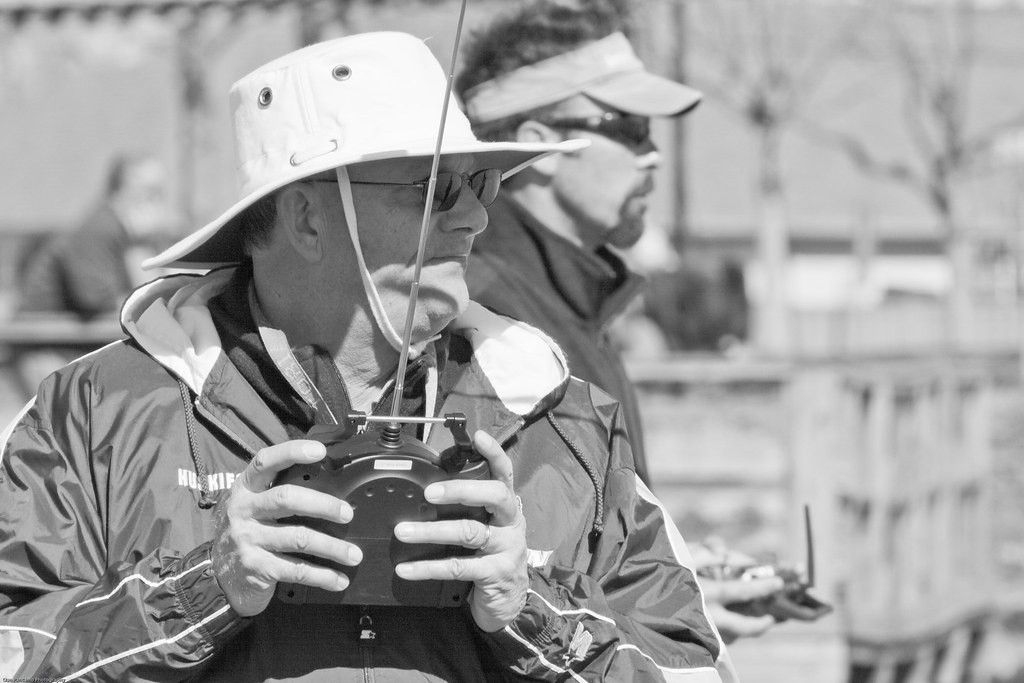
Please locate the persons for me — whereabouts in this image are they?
[0,29,736,683]
[447,0,818,646]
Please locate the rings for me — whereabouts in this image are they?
[477,522,491,551]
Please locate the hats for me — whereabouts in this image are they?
[460,29,705,126]
[141,31,593,272]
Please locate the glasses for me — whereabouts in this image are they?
[304,168,505,213]
[543,114,650,147]
